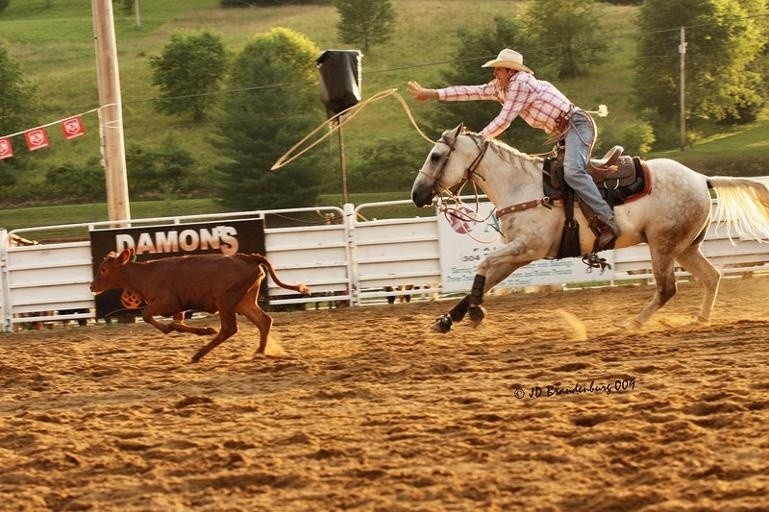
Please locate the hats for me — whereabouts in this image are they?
[482,49,534,75]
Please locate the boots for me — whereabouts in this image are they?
[599,219,618,246]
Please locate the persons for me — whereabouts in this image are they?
[407,49,620,251]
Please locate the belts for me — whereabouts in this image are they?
[558,107,578,131]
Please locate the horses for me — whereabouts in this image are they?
[410,122,769,334]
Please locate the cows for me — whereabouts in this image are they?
[89,247,311,364]
